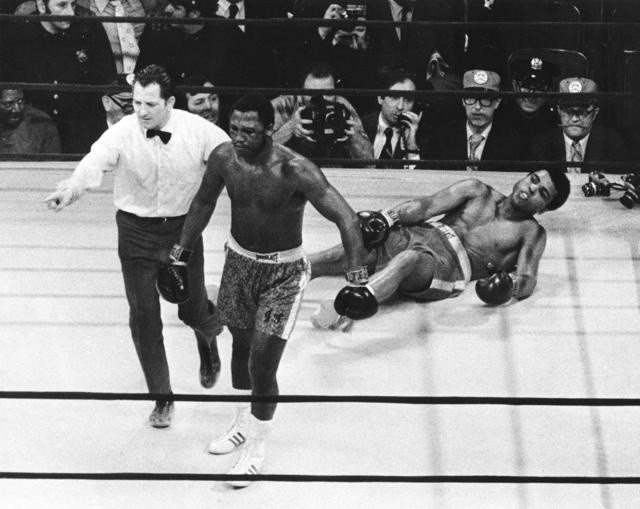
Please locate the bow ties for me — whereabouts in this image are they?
[147,129,171,144]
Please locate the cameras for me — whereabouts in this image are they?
[583,170,610,196]
[619,169,640,208]
[299,95,351,150]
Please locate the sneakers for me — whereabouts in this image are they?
[209,423,250,454]
[229,452,266,486]
[310,298,341,330]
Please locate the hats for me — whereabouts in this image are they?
[558,77,599,105]
[509,58,559,85]
[463,69,501,92]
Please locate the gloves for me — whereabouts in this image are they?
[475,271,520,305]
[334,266,378,320]
[157,244,194,304]
[356,209,393,249]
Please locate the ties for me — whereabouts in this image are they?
[569,141,583,173]
[467,134,484,170]
[375,128,393,169]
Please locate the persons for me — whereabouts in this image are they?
[44,61,232,429]
[156,93,377,488]
[305,168,570,333]
[1,1,639,171]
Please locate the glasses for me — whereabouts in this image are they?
[107,95,133,115]
[559,107,596,118]
[516,81,549,93]
[462,98,498,107]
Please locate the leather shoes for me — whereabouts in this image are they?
[198,337,220,388]
[149,392,175,427]
